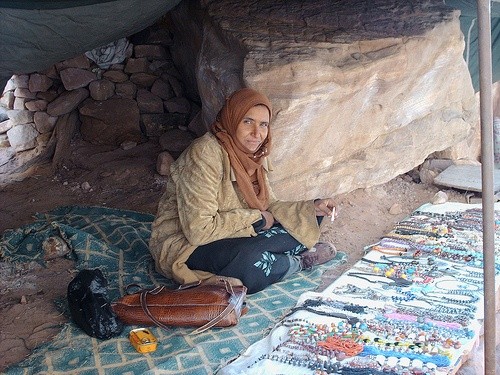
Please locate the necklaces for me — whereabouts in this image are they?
[241,204,500,375]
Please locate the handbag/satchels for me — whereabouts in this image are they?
[109,282,249,336]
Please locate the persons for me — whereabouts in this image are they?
[148,89,344,296]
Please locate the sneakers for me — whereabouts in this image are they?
[294,241,337,272]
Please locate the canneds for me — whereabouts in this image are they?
[129,327,158,353]
